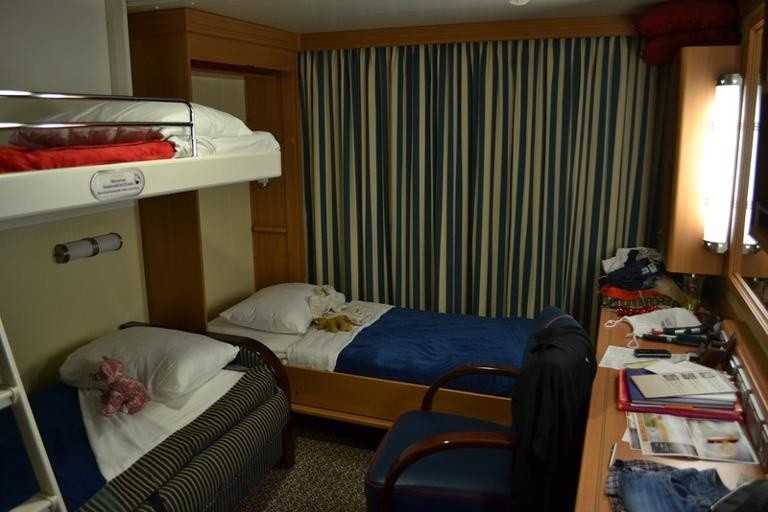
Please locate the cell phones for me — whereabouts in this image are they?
[634,348,671,357]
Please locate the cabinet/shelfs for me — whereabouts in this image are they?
[655,41,746,282]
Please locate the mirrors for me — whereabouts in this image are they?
[722,8,766,342]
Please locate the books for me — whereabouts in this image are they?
[616,365,744,421]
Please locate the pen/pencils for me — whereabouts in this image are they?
[705,436,739,443]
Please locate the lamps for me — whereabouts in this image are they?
[741,77,762,263]
[51,233,126,266]
[698,69,745,255]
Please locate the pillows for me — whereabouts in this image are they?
[218,282,347,337]
[14,96,253,145]
[57,321,238,408]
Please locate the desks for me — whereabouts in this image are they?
[570,285,766,512]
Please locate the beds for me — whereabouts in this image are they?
[206,296,536,442]
[0,89,281,233]
[0,313,299,512]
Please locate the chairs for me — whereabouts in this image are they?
[363,305,593,512]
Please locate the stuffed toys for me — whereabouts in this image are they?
[315,314,352,333]
[93,359,152,416]
